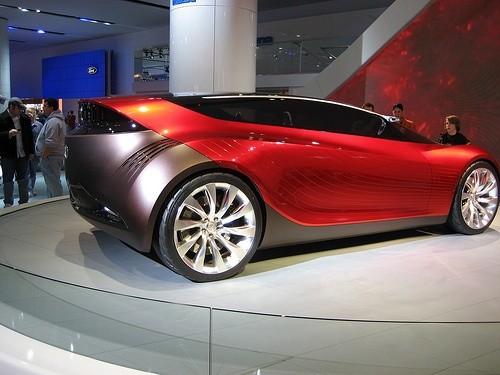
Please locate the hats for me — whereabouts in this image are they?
[8,97,27,110]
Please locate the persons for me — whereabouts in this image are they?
[392,102,417,136]
[439,115,472,146]
[66,110,76,131]
[37,112,46,125]
[25,105,43,197]
[0,97,35,208]
[35,98,66,198]
[362,102,374,111]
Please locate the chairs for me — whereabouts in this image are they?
[282,111,293,128]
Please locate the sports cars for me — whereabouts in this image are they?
[62,92,500,283]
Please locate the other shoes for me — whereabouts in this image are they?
[28,191,33,197]
[4,204,12,208]
